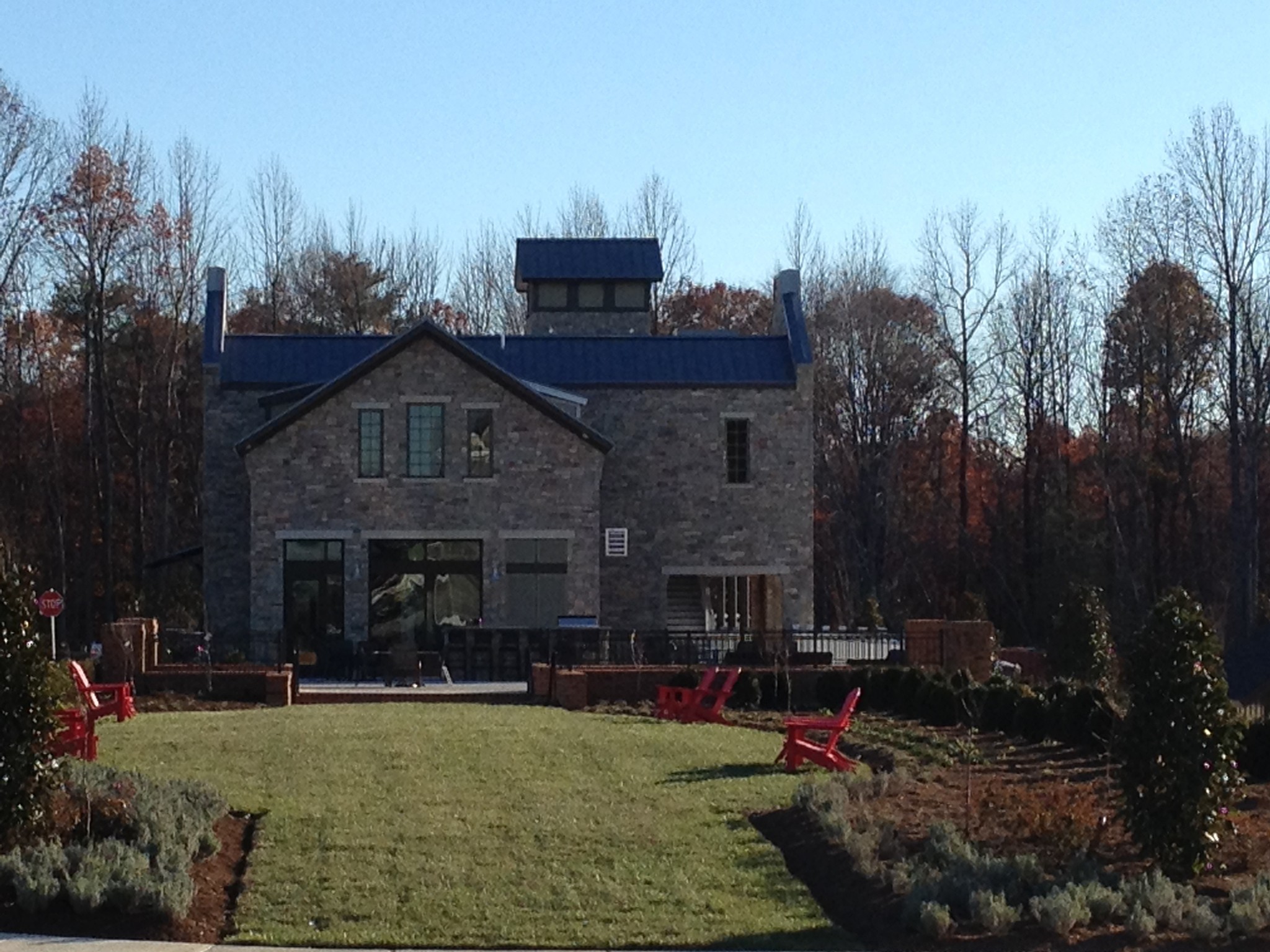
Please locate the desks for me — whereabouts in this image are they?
[444,625,614,679]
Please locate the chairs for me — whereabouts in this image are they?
[774,689,863,774]
[658,669,741,728]
[47,662,132,760]
[386,619,452,684]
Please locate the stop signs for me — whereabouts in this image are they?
[37,590,64,618]
